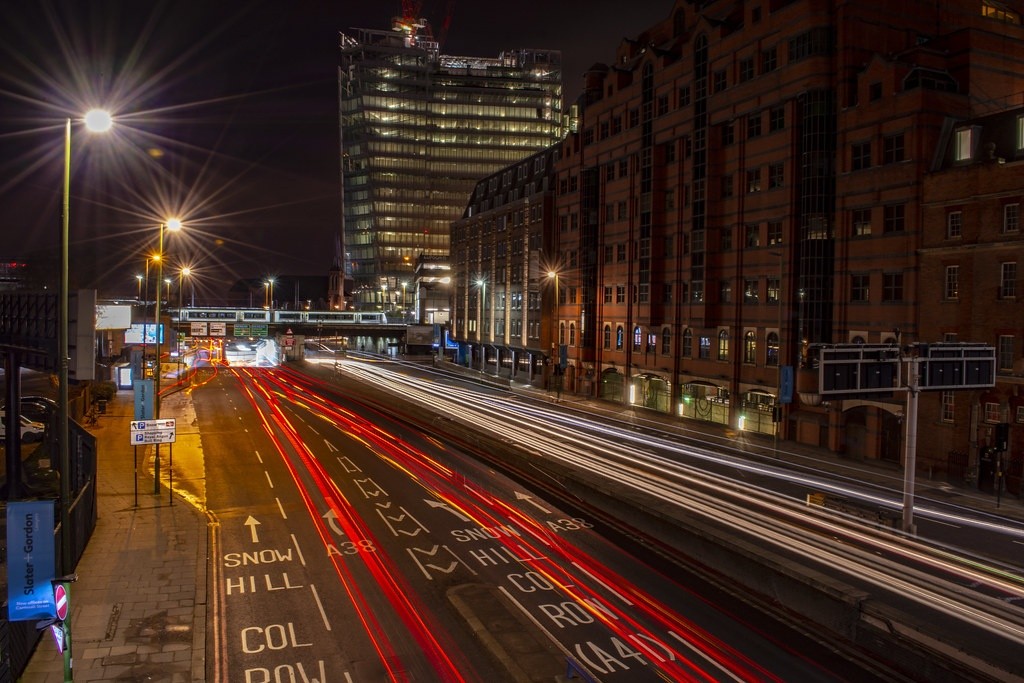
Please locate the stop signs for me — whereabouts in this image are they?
[55,584,70,622]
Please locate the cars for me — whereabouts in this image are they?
[21,396,57,406]
[0,402,47,421]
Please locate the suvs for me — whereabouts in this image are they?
[0,411,46,445]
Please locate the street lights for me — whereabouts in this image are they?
[401,282,407,317]
[137,275,143,301]
[381,284,387,312]
[154,218,183,495]
[141,254,161,417]
[177,267,191,383]
[269,279,274,309]
[548,271,560,391]
[61,107,115,680]
[164,279,171,303]
[264,282,269,309]
[476,279,486,369]
